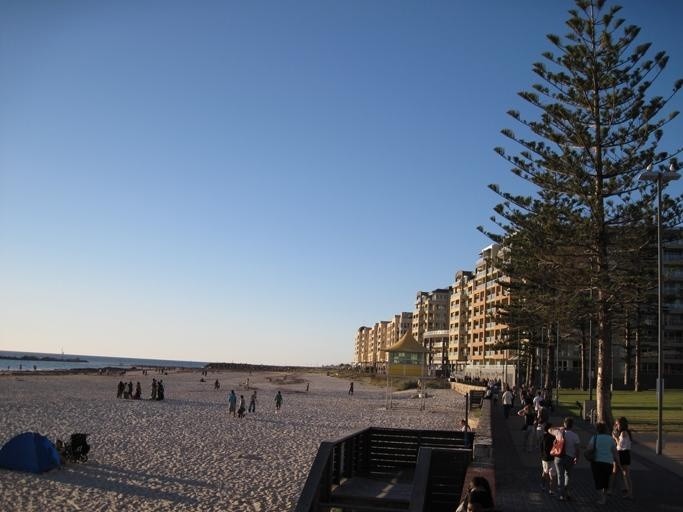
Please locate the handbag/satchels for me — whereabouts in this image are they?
[549,439,565,456]
[521,425,528,431]
[584,447,596,461]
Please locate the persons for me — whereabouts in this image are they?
[306,383,309,391]
[246,377,250,390]
[116,363,208,401]
[275,391,283,410]
[247,390,258,413]
[228,389,236,417]
[348,382,353,395]
[236,395,246,420]
[454,370,634,512]
[214,378,220,392]
[415,378,422,392]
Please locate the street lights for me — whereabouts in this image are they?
[637,160,681,456]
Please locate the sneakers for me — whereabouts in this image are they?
[539,486,570,500]
